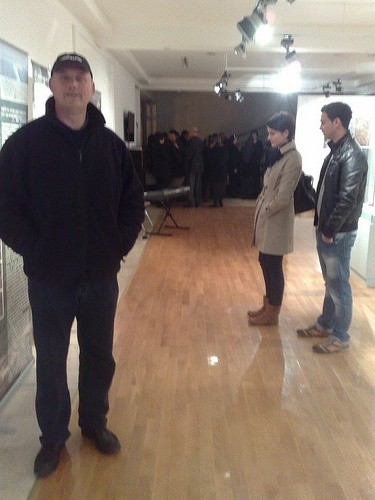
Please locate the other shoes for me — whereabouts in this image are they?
[81,418,122,455]
[296,326,333,337]
[312,335,350,354]
[33,435,65,477]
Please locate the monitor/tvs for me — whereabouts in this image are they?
[127,112,134,141]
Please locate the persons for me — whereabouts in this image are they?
[147,127,263,208]
[0,52,145,480]
[297,102,368,353]
[247,111,302,325]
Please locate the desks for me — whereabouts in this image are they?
[144,186,190,237]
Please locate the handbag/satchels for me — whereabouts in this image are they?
[294,172,317,214]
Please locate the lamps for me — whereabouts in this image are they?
[214,71,244,104]
[280,35,301,77]
[234,0,296,56]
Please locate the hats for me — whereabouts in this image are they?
[51,54,93,78]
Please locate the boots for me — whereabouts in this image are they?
[247,295,282,325]
